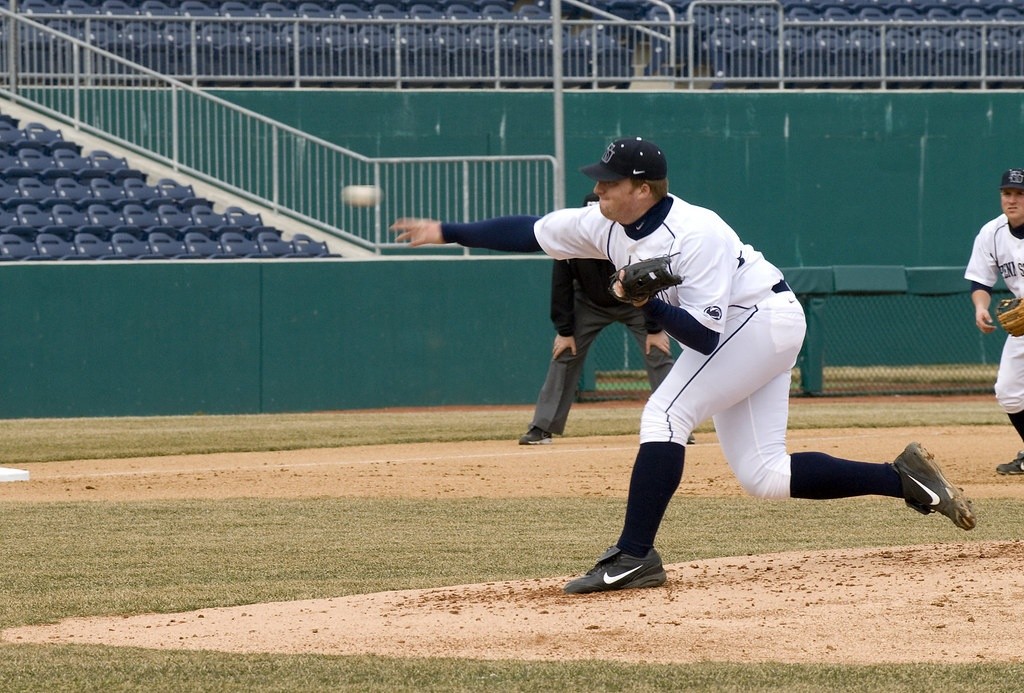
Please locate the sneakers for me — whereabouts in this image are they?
[686,436,695,444]
[894,441,977,531]
[564,546,667,594]
[996,451,1024,476]
[519,426,553,445]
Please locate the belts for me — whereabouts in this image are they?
[771,281,790,293]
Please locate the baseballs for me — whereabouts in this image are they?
[345,184,382,207]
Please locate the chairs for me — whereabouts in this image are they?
[0,0,1024,90]
[0,108,343,262]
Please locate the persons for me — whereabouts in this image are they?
[964,168,1024,476]
[519,190,698,445]
[389,136,977,595]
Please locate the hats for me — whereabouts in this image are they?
[998,167,1024,190]
[582,136,667,182]
[584,193,599,207]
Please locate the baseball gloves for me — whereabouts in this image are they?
[995,296,1024,338]
[608,251,686,311]
